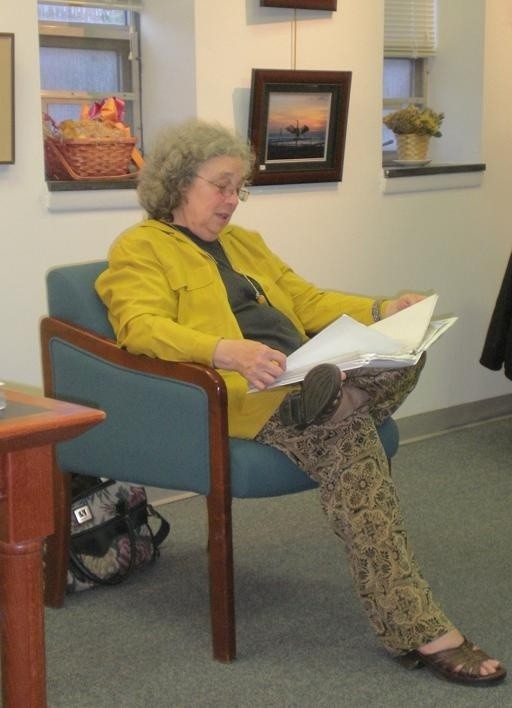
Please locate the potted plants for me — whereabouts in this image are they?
[383,103,445,167]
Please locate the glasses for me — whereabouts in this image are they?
[196,175,250,202]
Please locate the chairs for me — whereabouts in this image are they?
[41,259,399,663]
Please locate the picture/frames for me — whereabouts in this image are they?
[248,69,352,184]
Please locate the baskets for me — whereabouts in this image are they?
[44,136,144,180]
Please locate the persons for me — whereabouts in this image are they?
[94,121,507,684]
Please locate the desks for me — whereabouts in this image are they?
[1,387,105,702]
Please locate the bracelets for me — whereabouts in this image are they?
[372,298,386,321]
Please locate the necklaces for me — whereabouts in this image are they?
[204,240,265,304]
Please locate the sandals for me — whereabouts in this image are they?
[280,364,343,431]
[398,635,507,688]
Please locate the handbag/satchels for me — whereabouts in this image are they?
[42,472,169,596]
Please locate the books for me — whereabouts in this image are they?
[245,294,459,394]
